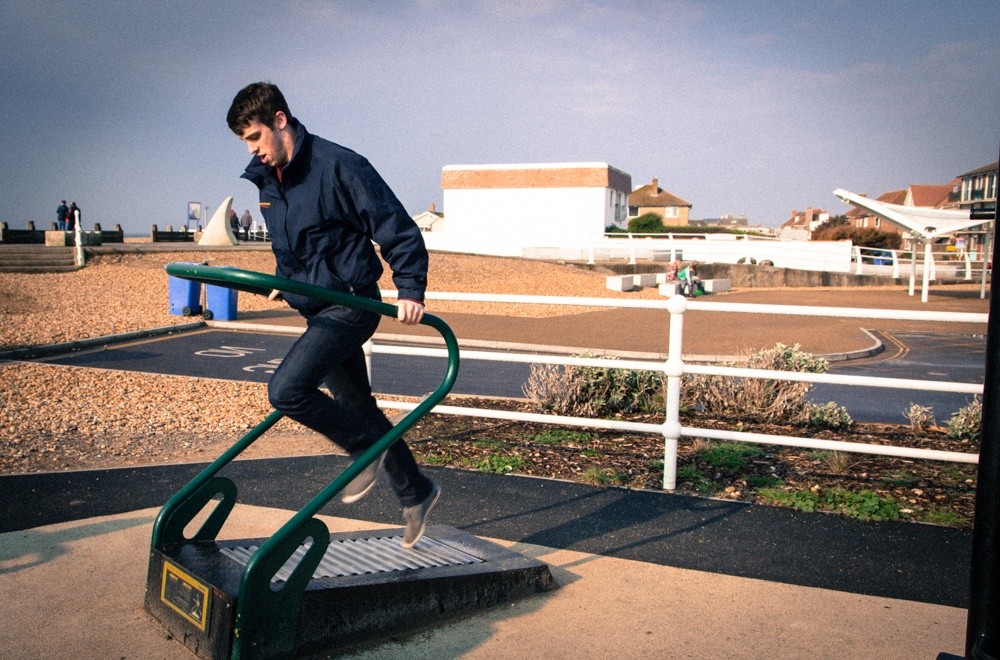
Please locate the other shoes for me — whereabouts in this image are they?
[402,484,442,547]
[342,446,388,503]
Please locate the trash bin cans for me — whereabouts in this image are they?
[203,266,240,321]
[163,261,208,316]
[873,251,892,265]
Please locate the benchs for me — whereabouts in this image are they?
[45,231,101,246]
[605,273,732,299]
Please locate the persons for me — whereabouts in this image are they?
[223,81,442,550]
[667,258,705,298]
[229,207,253,241]
[56,200,81,231]
